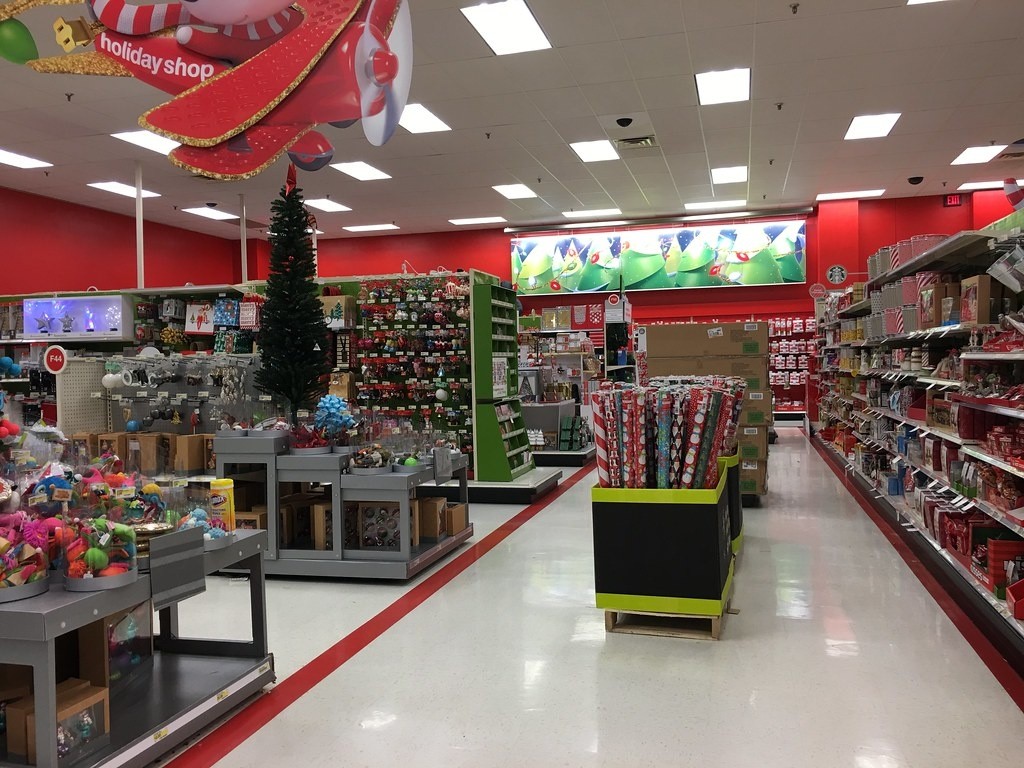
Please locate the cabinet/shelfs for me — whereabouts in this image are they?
[516,306,640,393]
[213,437,473,579]
[805,207,1018,563]
[767,317,818,420]
[592,444,747,614]
[0,530,275,768]
[0,353,278,435]
[0,267,562,504]
[931,207,1024,681]
[517,370,596,468]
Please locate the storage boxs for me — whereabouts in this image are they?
[822,273,1024,619]
[638,323,774,494]
[0,296,607,768]
